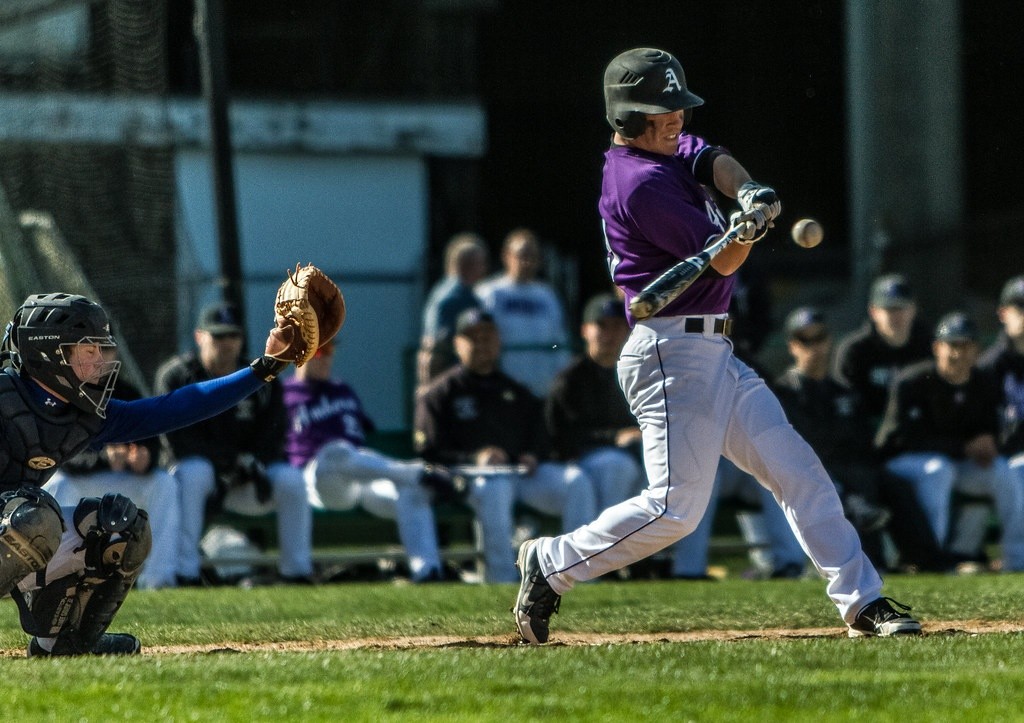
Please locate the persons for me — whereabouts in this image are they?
[413,232,661,582]
[512,48,921,643]
[41,306,446,587]
[0,266,345,660]
[671,275,1024,581]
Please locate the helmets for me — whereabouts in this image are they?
[11,292,122,419]
[604,47,703,140]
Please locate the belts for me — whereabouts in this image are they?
[685,316,734,337]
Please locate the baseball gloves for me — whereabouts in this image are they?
[264,265,347,369]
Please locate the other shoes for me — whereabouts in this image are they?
[845,493,889,530]
[422,465,467,497]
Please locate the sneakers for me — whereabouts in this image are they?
[848,597,921,638]
[27,632,141,659]
[511,539,561,645]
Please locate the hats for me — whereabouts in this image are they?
[198,301,242,331]
[1000,276,1024,308]
[933,311,978,343]
[583,295,623,322]
[868,275,915,309]
[457,311,491,334]
[785,308,823,333]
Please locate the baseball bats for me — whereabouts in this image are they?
[628,221,747,319]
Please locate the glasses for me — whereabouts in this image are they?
[211,331,243,341]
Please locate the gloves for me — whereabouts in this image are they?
[729,209,768,245]
[737,181,781,228]
[217,453,272,503]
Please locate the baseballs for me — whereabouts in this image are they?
[791,218,823,248]
